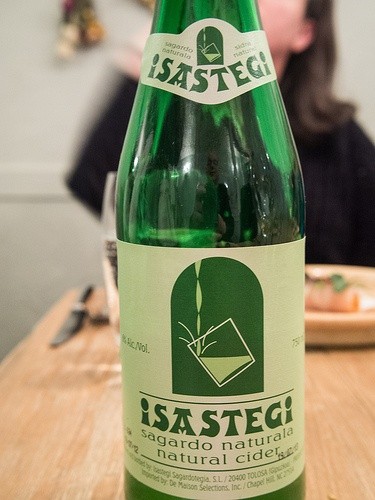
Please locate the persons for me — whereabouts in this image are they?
[66,0,375,268]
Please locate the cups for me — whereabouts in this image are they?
[104,175,123,327]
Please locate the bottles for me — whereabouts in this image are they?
[117,0,306,500]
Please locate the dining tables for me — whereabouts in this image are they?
[0,287,375,500]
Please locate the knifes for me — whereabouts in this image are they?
[49,285,97,347]
